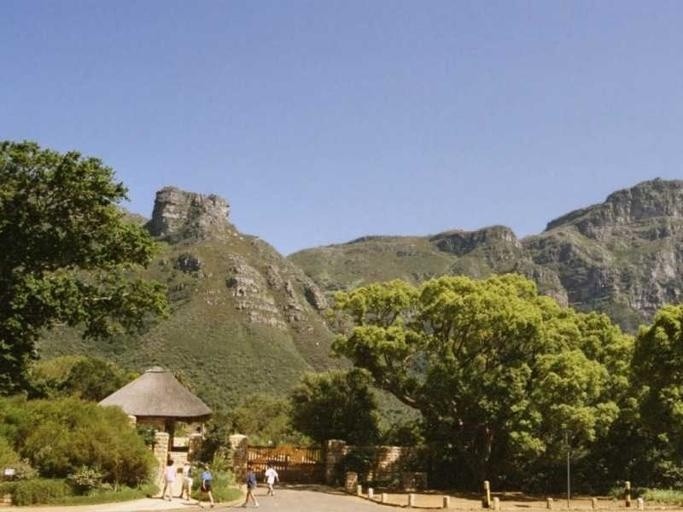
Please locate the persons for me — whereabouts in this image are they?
[178,461,197,500]
[161,460,177,501]
[197,462,216,507]
[263,464,281,496]
[238,467,260,508]
